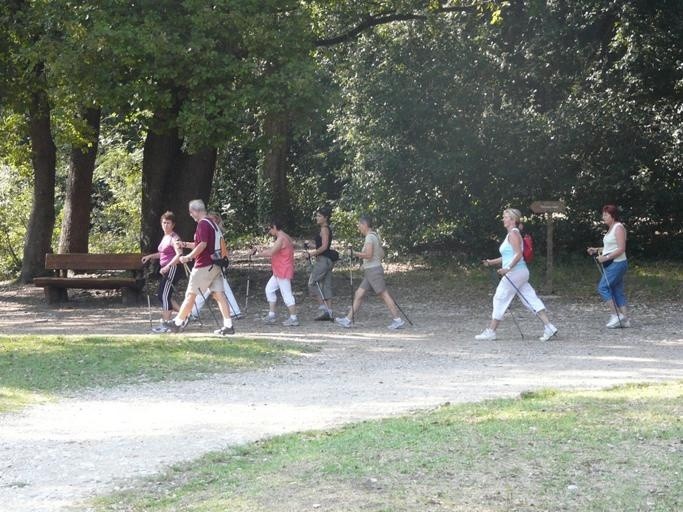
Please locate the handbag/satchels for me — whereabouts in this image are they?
[326,249,338,262]
[601,252,613,268]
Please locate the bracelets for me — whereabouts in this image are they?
[606,255,610,260]
[507,266,511,271]
[186,255,192,262]
[167,263,172,268]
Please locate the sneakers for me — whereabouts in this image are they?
[618,320,630,328]
[387,317,406,330]
[259,315,276,323]
[539,322,558,342]
[474,328,496,342]
[606,313,624,329]
[314,311,334,322]
[282,318,299,326]
[336,316,351,328]
[213,325,235,336]
[153,315,197,333]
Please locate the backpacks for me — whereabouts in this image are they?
[203,217,229,279]
[523,233,534,264]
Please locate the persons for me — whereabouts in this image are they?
[300,208,339,321]
[475,208,558,342]
[334,217,405,329]
[252,220,299,326]
[172,215,243,321]
[162,199,235,335]
[141,211,183,333]
[586,205,631,329]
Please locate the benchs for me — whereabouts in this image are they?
[33,248,152,308]
